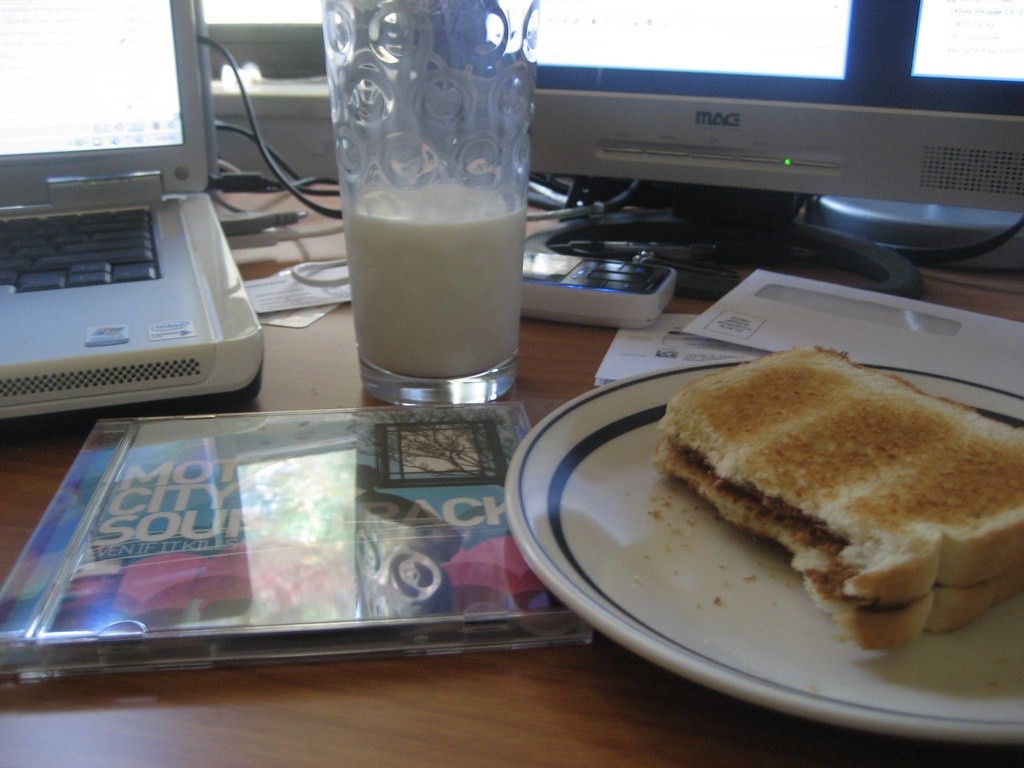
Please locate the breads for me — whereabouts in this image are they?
[654,344,1024,651]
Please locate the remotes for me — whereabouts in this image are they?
[519,253,677,327]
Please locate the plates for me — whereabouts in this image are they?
[505,363,1024,745]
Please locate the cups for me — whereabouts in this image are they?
[320,0,539,406]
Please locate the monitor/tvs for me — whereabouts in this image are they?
[434,0,1024,302]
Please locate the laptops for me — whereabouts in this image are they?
[0,0,262,421]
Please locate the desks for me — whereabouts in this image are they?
[0,183,1024,768]
